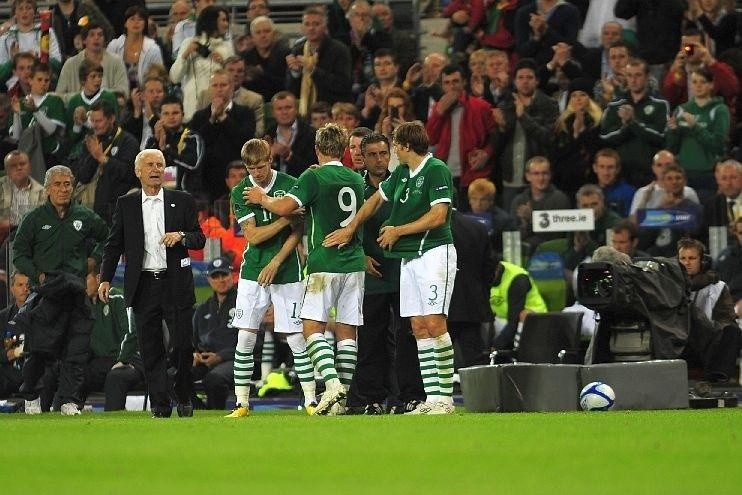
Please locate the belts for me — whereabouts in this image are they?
[141,271,166,279]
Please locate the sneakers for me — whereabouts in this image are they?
[405,401,437,415]
[692,381,712,397]
[25,395,42,415]
[403,400,419,411]
[61,403,81,417]
[225,403,249,417]
[388,406,403,413]
[363,404,384,415]
[313,383,346,416]
[428,402,455,415]
[303,401,318,415]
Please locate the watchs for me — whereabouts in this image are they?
[178,231,185,239]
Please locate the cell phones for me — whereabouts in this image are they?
[390,105,399,120]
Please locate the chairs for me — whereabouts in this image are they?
[489,312,584,366]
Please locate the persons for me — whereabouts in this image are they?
[0,93,18,172]
[565,183,622,272]
[489,259,549,359]
[240,121,369,416]
[610,218,651,260]
[193,55,265,138]
[0,0,62,92]
[575,0,637,77]
[374,87,429,179]
[284,6,352,116]
[468,48,488,79]
[331,102,361,170]
[225,138,321,418]
[171,0,216,63]
[490,59,554,216]
[513,0,579,66]
[249,297,301,397]
[326,0,354,45]
[96,149,206,418]
[7,62,67,165]
[717,215,742,317]
[474,0,521,86]
[55,22,130,110]
[469,50,512,108]
[210,160,248,238]
[676,236,742,383]
[169,5,235,124]
[83,258,146,412]
[467,48,488,76]
[5,51,56,99]
[144,63,184,103]
[450,187,498,366]
[122,75,167,150]
[348,126,374,179]
[48,0,116,62]
[0,151,44,284]
[340,0,394,93]
[233,0,290,56]
[113,92,126,120]
[403,53,448,126]
[167,257,237,409]
[0,270,31,397]
[434,0,488,34]
[186,68,255,204]
[613,0,690,91]
[659,28,742,128]
[322,119,459,413]
[354,131,425,414]
[597,57,670,190]
[147,96,206,192]
[698,159,742,252]
[628,148,700,219]
[581,21,623,82]
[258,90,319,180]
[75,100,139,233]
[550,76,603,186]
[466,178,517,256]
[664,67,730,188]
[355,47,403,132]
[592,40,659,111]
[13,164,109,417]
[309,101,333,129]
[423,63,497,213]
[591,148,638,219]
[537,40,593,98]
[638,164,706,260]
[509,156,570,259]
[164,0,192,64]
[106,5,164,91]
[238,16,290,101]
[371,3,418,81]
[66,57,118,165]
[684,0,742,61]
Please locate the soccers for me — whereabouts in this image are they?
[580,382,616,410]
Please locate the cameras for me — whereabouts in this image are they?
[194,41,211,59]
[684,42,694,55]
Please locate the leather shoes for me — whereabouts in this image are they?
[153,412,171,417]
[176,395,193,417]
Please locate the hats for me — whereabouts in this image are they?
[569,77,594,98]
[206,258,234,276]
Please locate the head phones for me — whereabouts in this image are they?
[699,247,712,272]
